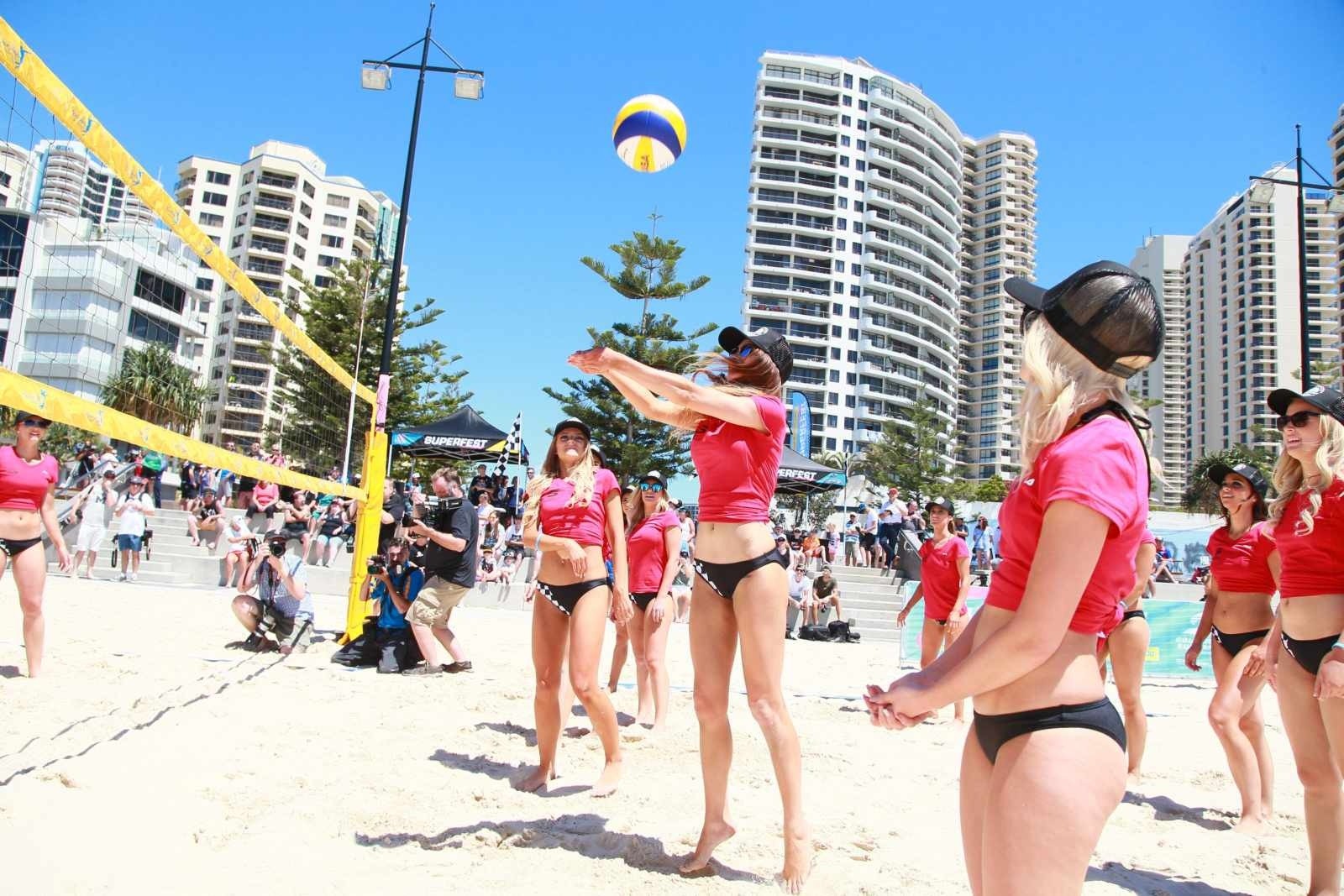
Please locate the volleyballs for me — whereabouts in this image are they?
[612,93,686,173]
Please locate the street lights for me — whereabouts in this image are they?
[1249,123,1344,394]
[360,1,486,432]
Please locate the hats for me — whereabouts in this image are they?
[590,444,605,467]
[718,325,794,385]
[104,470,115,477]
[638,470,666,488]
[553,417,591,441]
[925,497,954,513]
[1207,463,1268,498]
[778,537,785,542]
[264,530,286,543]
[1002,259,1166,379]
[16,413,34,424]
[1267,384,1344,426]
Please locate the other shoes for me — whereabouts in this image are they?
[440,660,473,674]
[316,560,324,566]
[402,660,445,677]
[325,562,330,567]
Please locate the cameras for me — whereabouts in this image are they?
[268,541,286,557]
[244,607,280,648]
[367,555,386,574]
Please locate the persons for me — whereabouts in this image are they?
[0,260,1344,896]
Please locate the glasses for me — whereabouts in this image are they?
[796,569,803,572]
[1020,304,1043,336]
[640,483,663,493]
[1277,411,1318,431]
[24,419,47,428]
[850,516,855,517]
[795,530,800,532]
[107,476,114,479]
[729,346,754,357]
